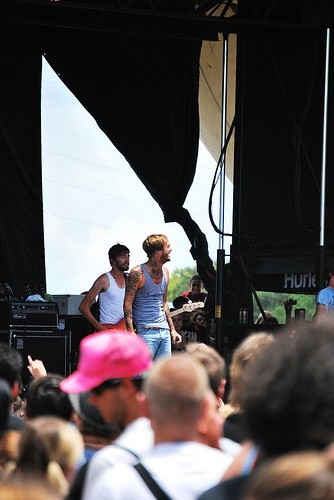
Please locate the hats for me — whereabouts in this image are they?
[59,330,152,394]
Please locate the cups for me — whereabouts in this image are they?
[59,319,65,330]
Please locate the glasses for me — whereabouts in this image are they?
[173,318,183,322]
[196,318,207,322]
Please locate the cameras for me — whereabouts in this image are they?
[286,299,297,307]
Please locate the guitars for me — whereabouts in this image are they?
[169,301,205,317]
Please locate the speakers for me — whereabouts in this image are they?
[0,329,72,389]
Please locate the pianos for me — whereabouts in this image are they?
[0,301,59,316]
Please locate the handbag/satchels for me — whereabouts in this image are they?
[79,302,100,334]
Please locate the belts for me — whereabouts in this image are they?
[141,327,164,330]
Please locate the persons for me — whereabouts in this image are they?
[171,310,215,353]
[80,273,280,325]
[311,262,334,321]
[0,312,334,500]
[124,234,183,360]
[77,243,129,332]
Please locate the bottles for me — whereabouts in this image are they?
[243,308,248,324]
[239,308,243,323]
